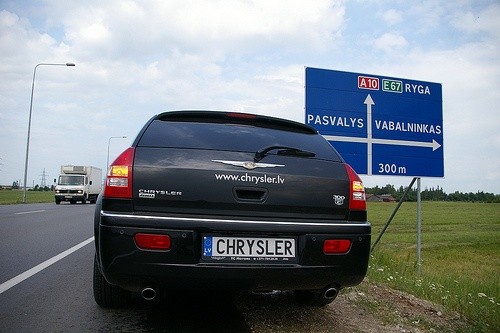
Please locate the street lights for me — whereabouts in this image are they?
[107,136,128,174]
[22,63,76,203]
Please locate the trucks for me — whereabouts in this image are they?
[55,164,102,204]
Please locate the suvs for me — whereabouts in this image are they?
[93,110,371,309]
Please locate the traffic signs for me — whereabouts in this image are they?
[306,66,444,176]
